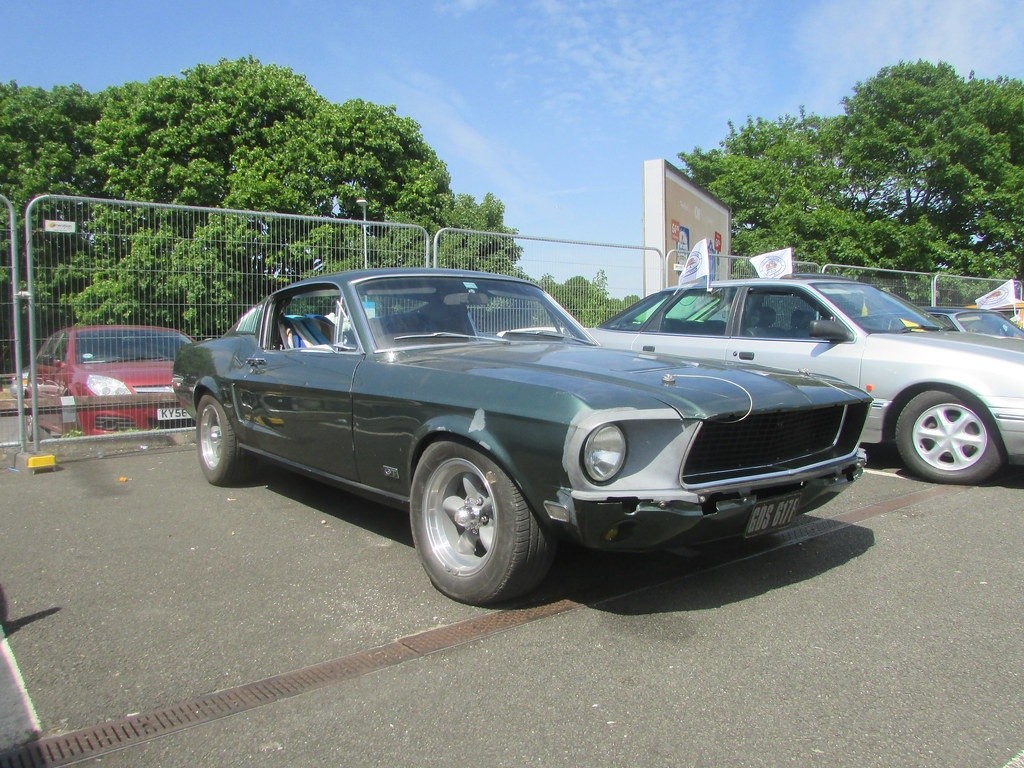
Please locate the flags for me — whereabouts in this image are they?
[673,240,708,295]
[749,248,792,278]
[975,280,1013,310]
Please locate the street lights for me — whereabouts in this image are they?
[356,199,368,269]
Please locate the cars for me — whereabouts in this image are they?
[10,371,28,399]
[854,306,1024,341]
[497,275,1024,485]
[36,380,62,396]
[24,325,201,438]
[173,269,873,607]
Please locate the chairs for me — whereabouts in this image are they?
[744,306,788,338]
[789,309,816,338]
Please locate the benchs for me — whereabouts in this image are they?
[662,318,726,336]
